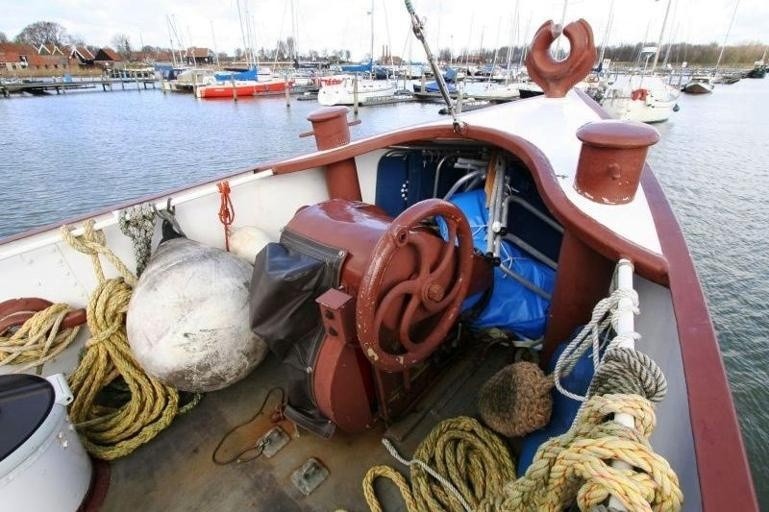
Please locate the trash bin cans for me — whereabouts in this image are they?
[0,374,92,511]
